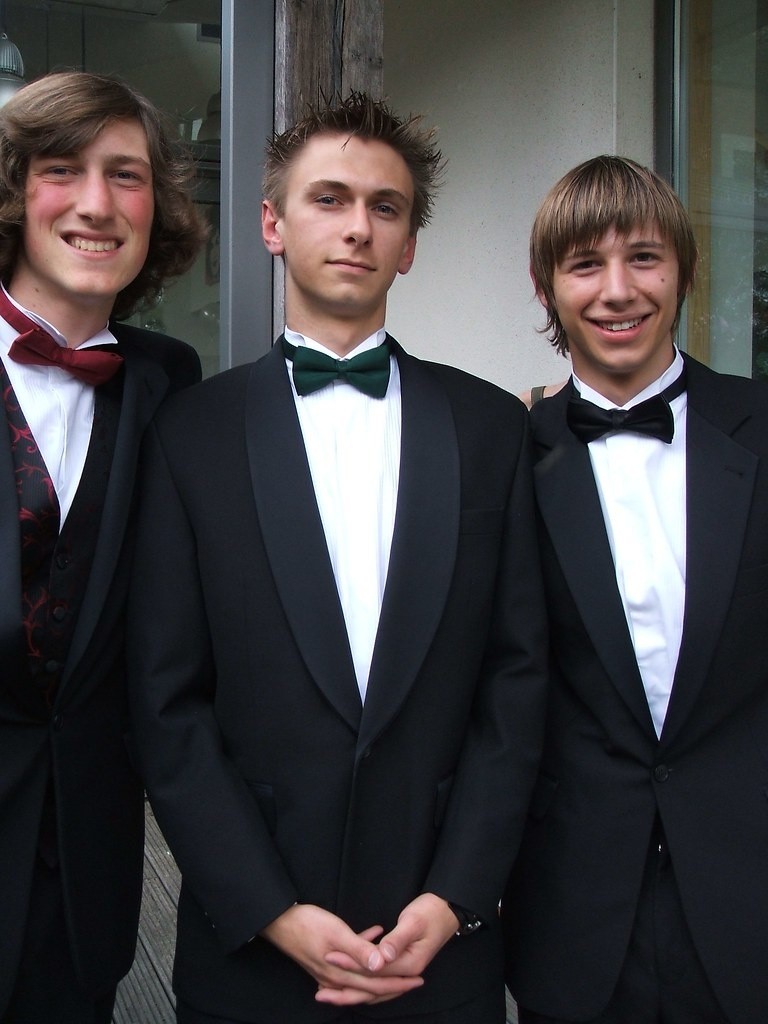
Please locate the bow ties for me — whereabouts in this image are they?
[8,328,123,386]
[292,346,391,398]
[566,392,674,445]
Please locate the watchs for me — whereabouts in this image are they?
[447,900,481,939]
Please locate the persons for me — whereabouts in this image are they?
[0,71,211,1024]
[498,151,768,1024]
[140,92,535,1023]
